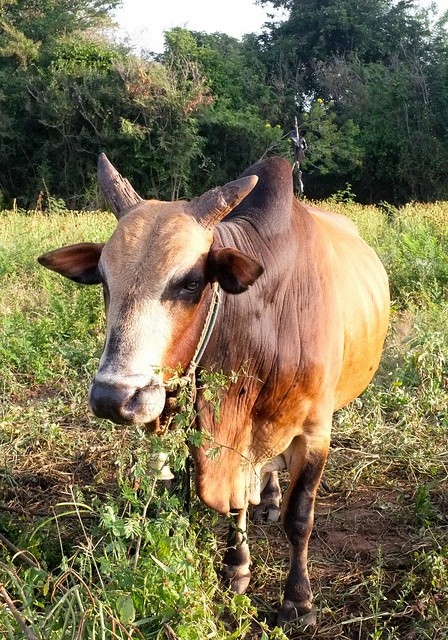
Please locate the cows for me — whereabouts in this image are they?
[37,152,390,615]
